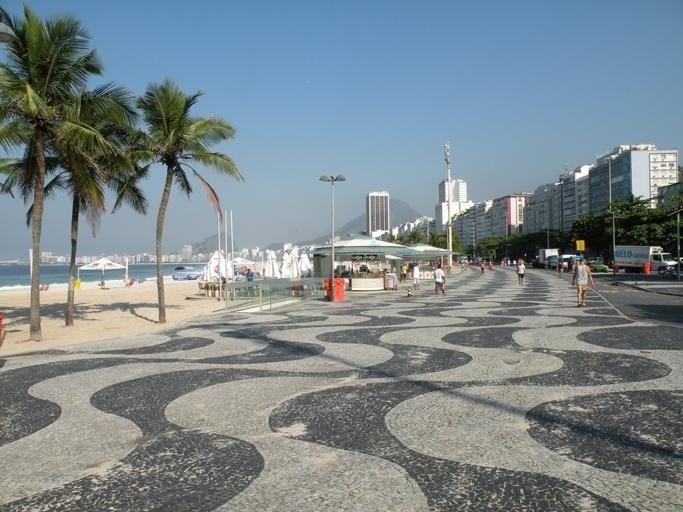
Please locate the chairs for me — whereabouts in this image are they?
[198,282,219,296]
[39,278,135,291]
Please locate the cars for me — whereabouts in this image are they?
[589,262,609,273]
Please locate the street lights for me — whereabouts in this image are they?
[442,142,453,277]
[321,174,347,301]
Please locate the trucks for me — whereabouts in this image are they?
[538,248,560,269]
[615,244,677,275]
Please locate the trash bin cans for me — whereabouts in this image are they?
[384,273,397,290]
[644,262,651,274]
[325,278,345,302]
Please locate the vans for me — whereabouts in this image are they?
[558,254,577,272]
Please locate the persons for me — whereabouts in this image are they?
[400,262,420,291]
[431,254,493,295]
[557,257,593,307]
[239,266,253,280]
[500,257,526,286]
